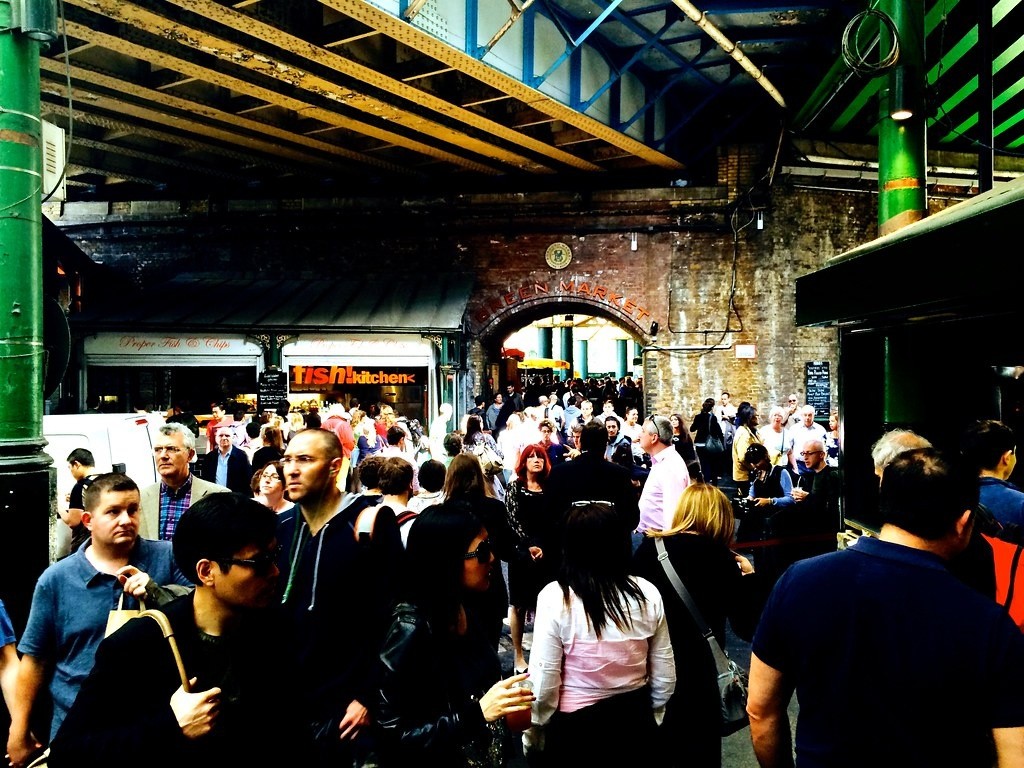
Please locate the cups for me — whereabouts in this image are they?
[506,681,536,731]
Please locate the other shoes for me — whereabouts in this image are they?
[513,660,529,676]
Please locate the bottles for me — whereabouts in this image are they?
[319,400,338,408]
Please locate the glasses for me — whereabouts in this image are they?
[280,453,337,467]
[216,544,282,575]
[801,450,823,457]
[650,413,660,439]
[789,400,796,402]
[748,446,758,453]
[450,535,493,563]
[258,473,281,483]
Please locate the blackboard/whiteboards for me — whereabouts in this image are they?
[805,361,831,416]
[256,371,288,413]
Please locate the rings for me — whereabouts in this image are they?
[136,581,140,586]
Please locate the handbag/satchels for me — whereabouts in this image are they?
[706,435,725,453]
[714,658,751,737]
[104,588,148,637]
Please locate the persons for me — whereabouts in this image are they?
[745,419,1024,768]
[0,375,840,768]
